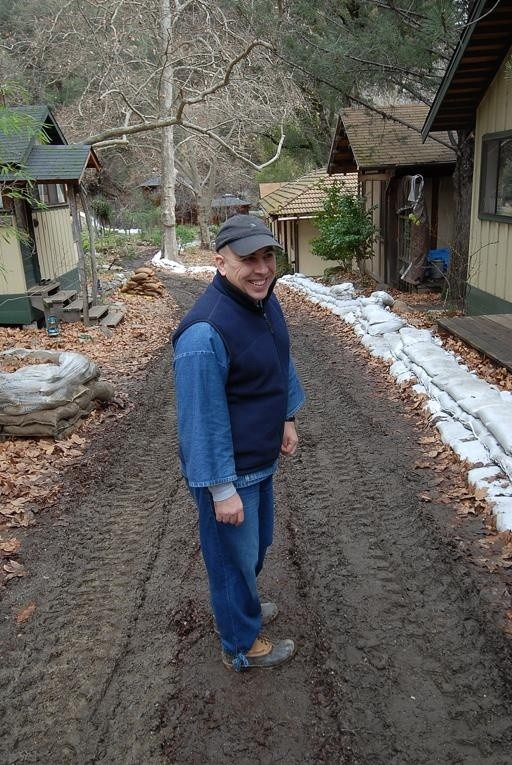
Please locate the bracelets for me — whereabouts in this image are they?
[285,415,295,422]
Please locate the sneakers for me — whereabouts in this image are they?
[221,637,297,669]
[213,603,279,635]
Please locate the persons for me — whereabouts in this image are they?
[171,214,306,673]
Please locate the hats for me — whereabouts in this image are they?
[215,214,284,257]
